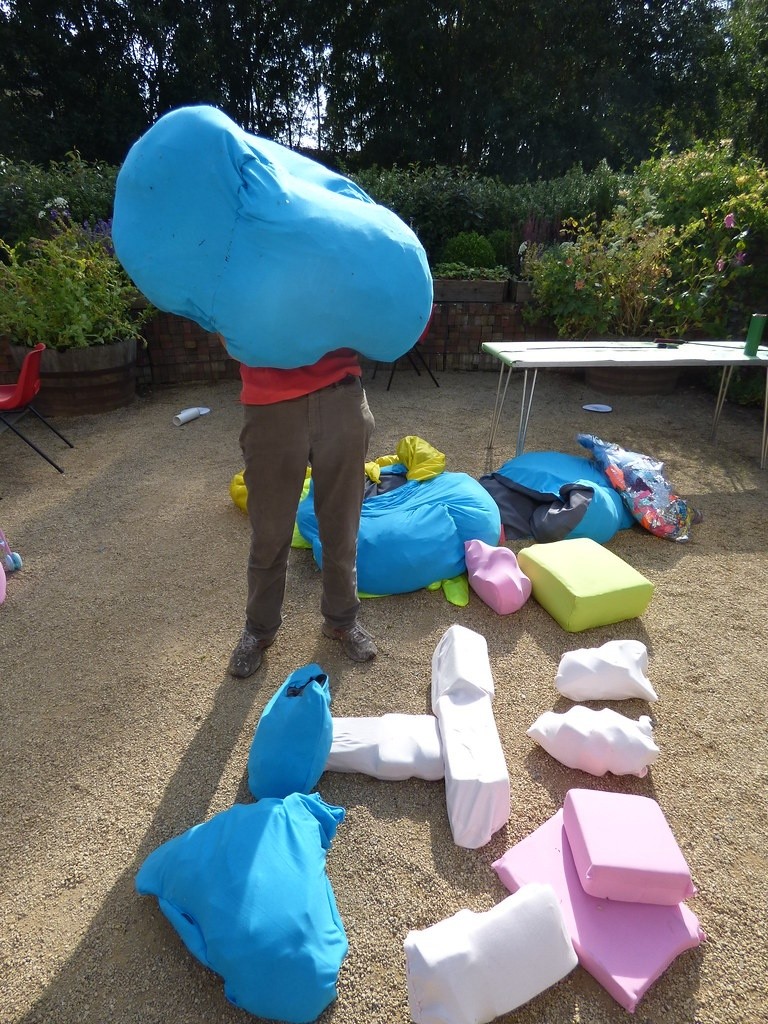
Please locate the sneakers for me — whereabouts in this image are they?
[319,618,379,664]
[226,626,277,679]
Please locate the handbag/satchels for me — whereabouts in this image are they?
[243,655,338,804]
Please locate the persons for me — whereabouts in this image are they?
[226,333,378,679]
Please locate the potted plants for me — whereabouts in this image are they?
[432,262,512,302]
[0,222,158,416]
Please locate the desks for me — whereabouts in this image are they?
[481,340,768,470]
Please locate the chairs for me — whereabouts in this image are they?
[0,342,74,474]
[372,305,441,392]
